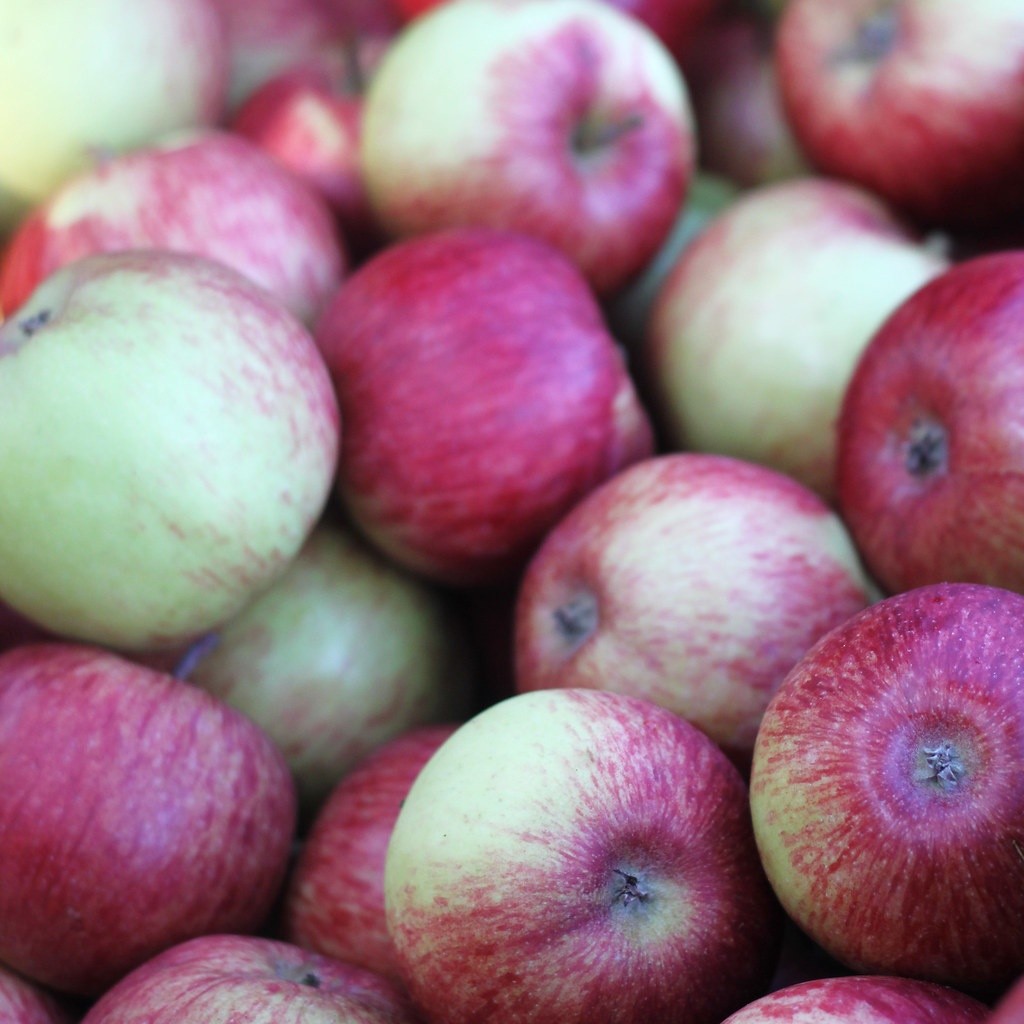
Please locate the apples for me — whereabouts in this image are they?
[0,0,1024,1024]
